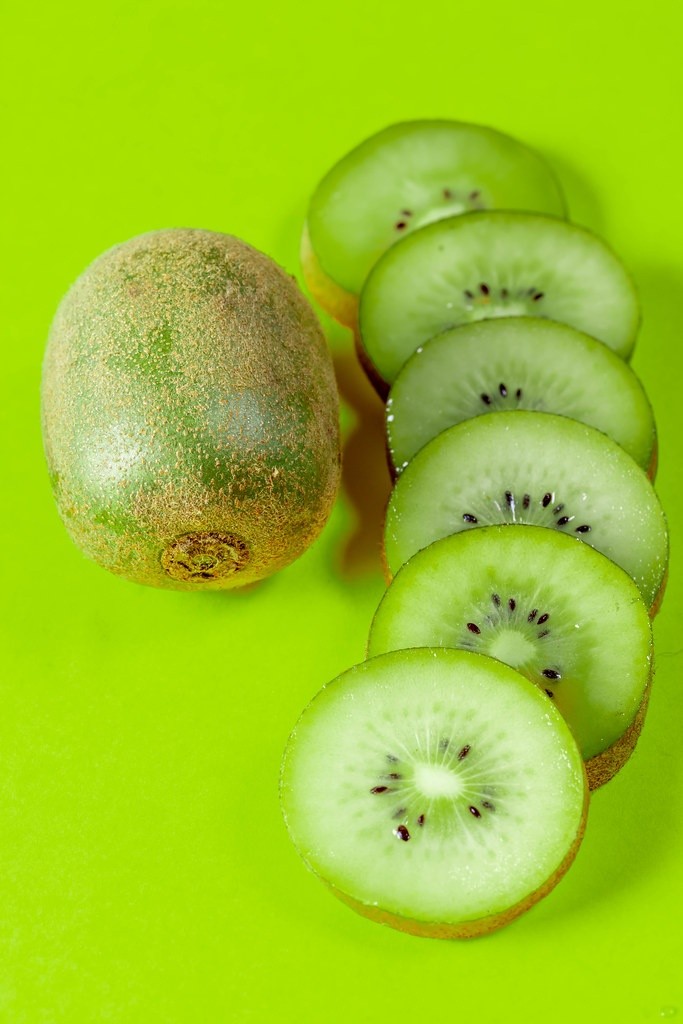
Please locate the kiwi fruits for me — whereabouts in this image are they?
[44,232,342,590]
[280,116,670,938]
